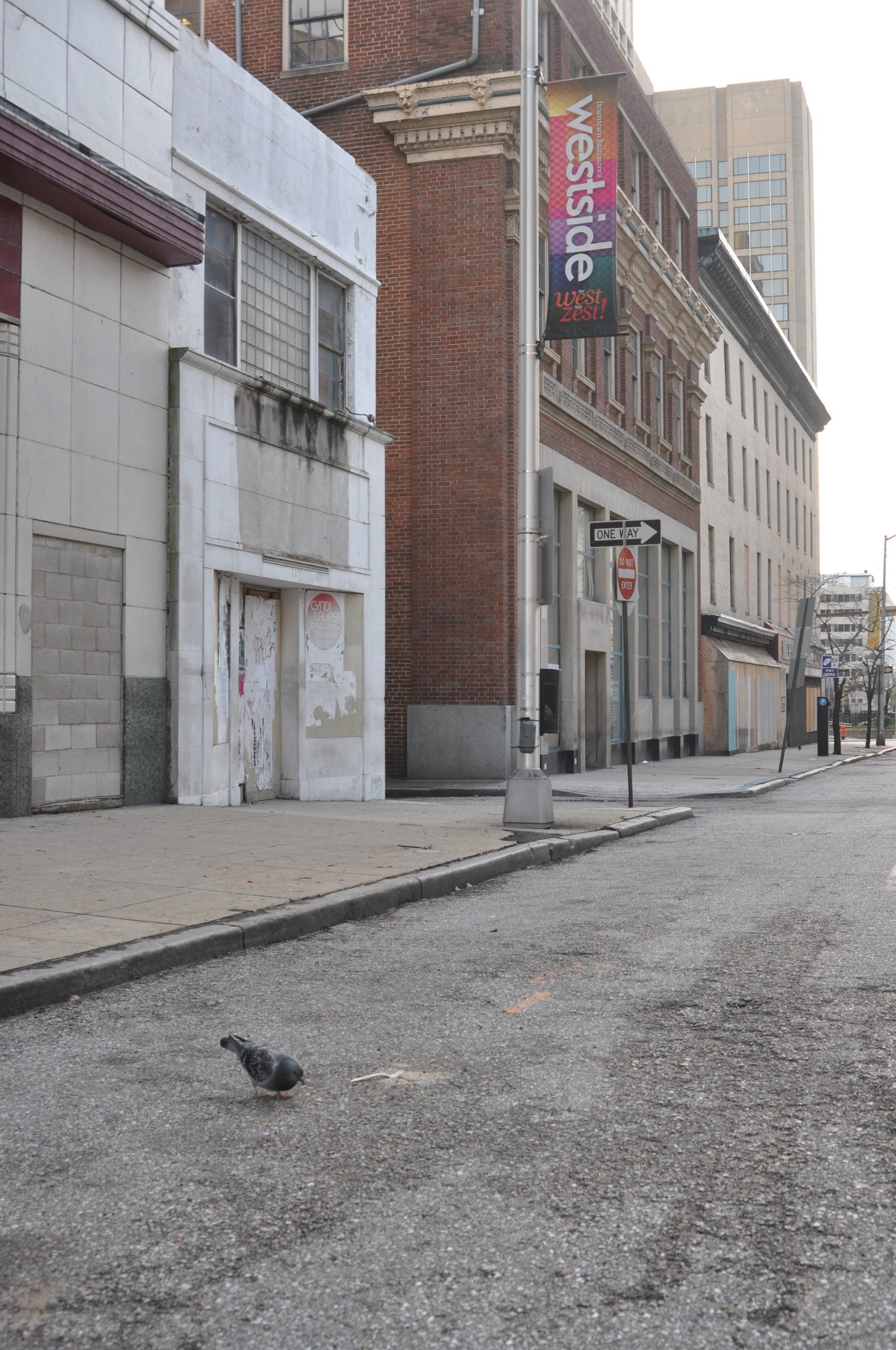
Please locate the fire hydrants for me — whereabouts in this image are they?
[839,725,847,740]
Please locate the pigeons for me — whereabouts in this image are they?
[220,1034,306,1099]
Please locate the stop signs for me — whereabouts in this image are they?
[617,547,637,601]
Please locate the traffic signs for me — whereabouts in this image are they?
[589,519,662,547]
[873,666,893,674]
